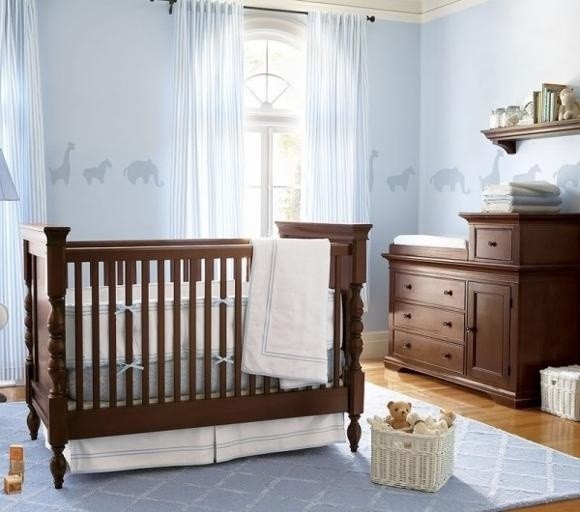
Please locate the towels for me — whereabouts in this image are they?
[394,234,468,248]
[482,182,562,212]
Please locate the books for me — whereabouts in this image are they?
[533,81,567,125]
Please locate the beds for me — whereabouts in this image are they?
[20,219,378,489]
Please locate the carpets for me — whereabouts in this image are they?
[0,379,580,512]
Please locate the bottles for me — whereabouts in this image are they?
[490,106,525,128]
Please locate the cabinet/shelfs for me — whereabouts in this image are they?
[381,211,580,409]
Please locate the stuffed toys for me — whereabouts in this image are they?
[366,399,457,433]
[557,88,580,121]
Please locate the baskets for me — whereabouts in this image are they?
[370,419,456,492]
[539,365,580,421]
